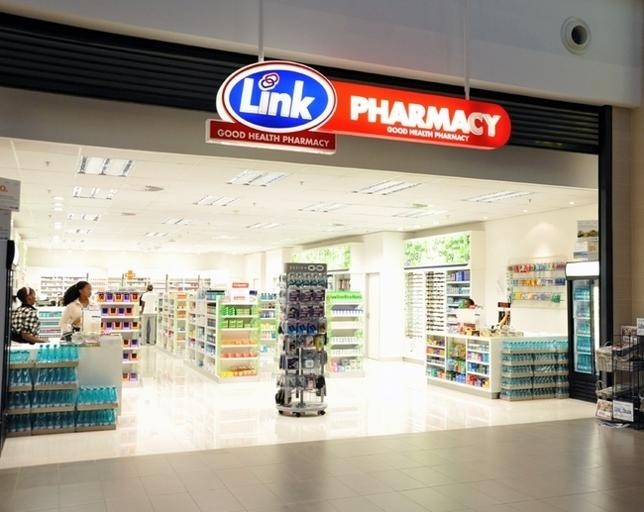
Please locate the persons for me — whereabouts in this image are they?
[141,285,157,344]
[59,281,96,341]
[139,296,154,343]
[11,287,48,344]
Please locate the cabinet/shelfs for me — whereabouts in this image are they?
[426,331,524,401]
[94,286,366,385]
[505,261,568,309]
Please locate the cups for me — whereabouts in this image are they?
[97,292,139,381]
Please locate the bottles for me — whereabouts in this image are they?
[326,290,362,299]
[499,338,568,397]
[7,343,119,434]
[572,287,594,372]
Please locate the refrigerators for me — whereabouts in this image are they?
[563,260,602,403]
